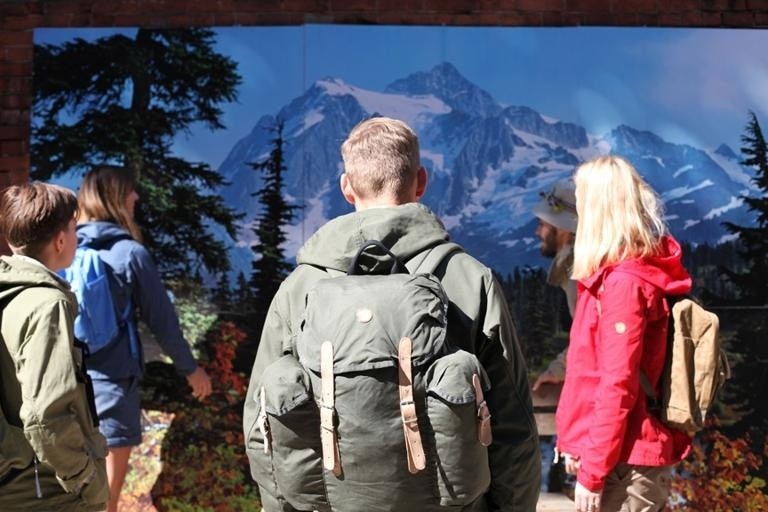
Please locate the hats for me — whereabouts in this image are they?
[532,184,578,234]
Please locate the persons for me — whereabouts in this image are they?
[551,153,695,511]
[240,118,546,512]
[527,173,586,502]
[56,164,214,512]
[0,179,113,511]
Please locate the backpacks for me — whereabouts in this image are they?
[245,240,494,511]
[638,294,731,433]
[55,246,136,363]
